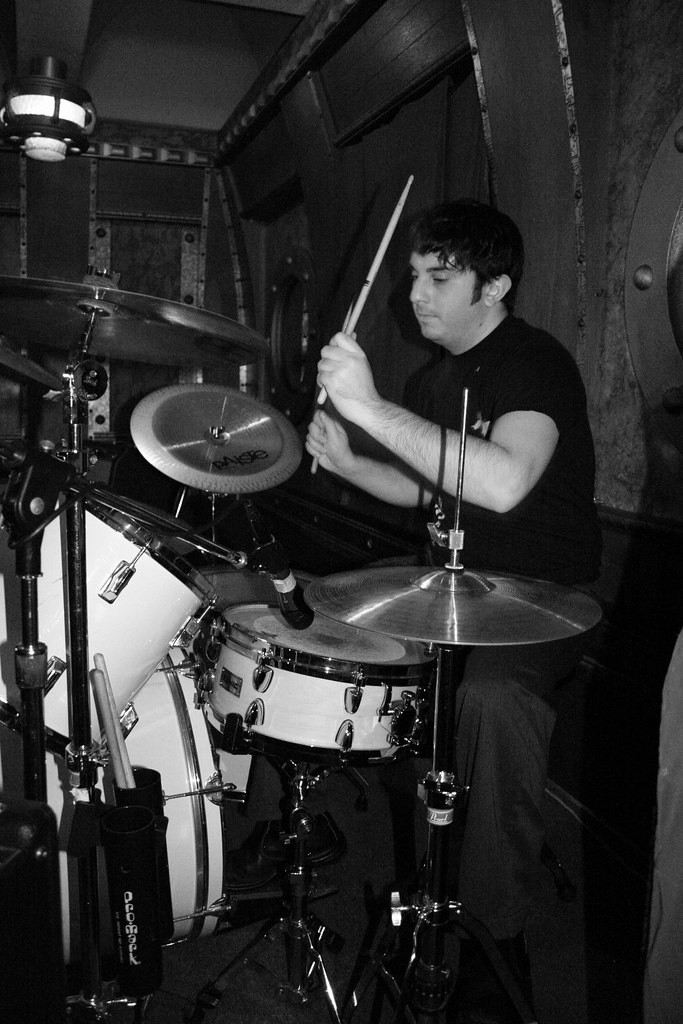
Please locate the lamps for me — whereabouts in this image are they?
[0,55,96,162]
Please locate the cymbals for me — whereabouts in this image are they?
[0,342,66,392]
[305,564,603,645]
[130,382,304,494]
[1,267,272,370]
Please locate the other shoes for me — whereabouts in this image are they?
[223,809,346,890]
[451,931,536,1024]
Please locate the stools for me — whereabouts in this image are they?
[539,679,592,902]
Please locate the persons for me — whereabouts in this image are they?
[223,197,599,1024]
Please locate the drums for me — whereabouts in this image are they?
[31,643,238,940]
[200,601,437,770]
[188,561,318,667]
[1,482,218,773]
